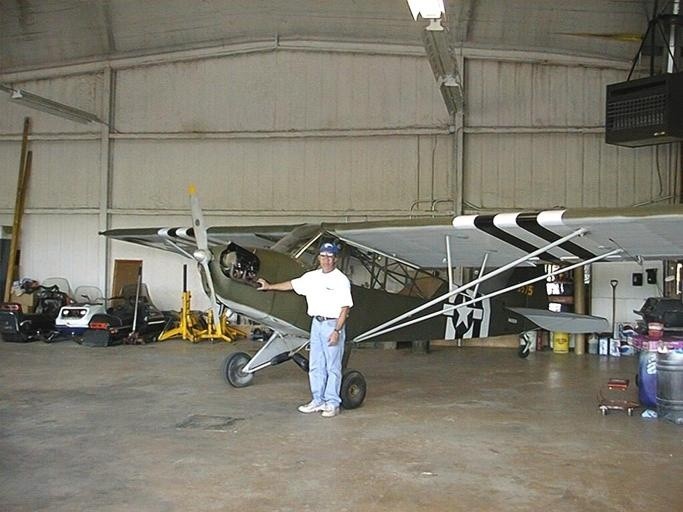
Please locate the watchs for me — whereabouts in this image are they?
[334,328,341,334]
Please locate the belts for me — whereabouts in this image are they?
[314,315,337,323]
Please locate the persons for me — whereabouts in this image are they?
[256,242,353,416]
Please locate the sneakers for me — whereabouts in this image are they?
[298,399,326,414]
[321,403,341,418]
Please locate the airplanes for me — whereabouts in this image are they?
[98,180,683,411]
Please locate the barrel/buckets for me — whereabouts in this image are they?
[588,333,598,354]
[655,344,683,424]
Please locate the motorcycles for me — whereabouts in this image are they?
[1,277,162,346]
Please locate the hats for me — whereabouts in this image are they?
[319,243,338,257]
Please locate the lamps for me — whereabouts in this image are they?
[5,88,100,128]
[420,19,459,83]
[404,0,447,24]
[438,80,470,117]
